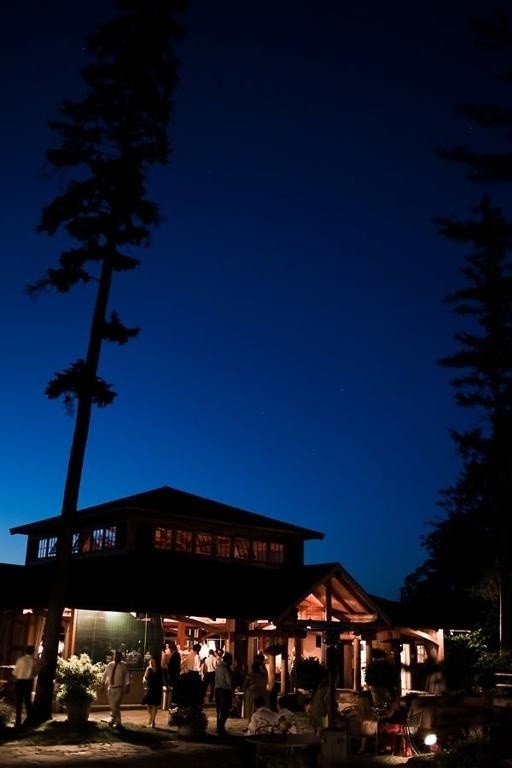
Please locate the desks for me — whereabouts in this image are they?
[243,733,322,768]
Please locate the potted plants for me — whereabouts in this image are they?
[50,652,111,723]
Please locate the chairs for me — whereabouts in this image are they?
[366,697,419,757]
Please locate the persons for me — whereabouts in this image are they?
[161,640,268,731]
[102,651,130,728]
[309,672,340,738]
[12,644,40,727]
[408,659,473,732]
[355,686,403,756]
[141,655,170,729]
[214,651,236,737]
